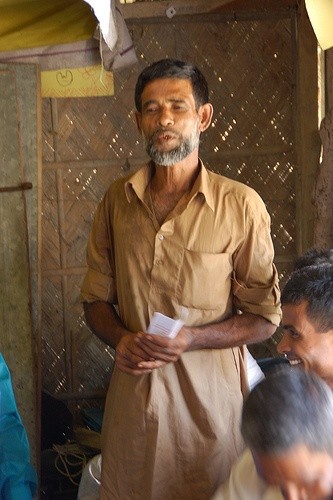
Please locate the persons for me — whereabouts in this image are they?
[277,263,333,385]
[213,368,333,500]
[81,59,282,500]
